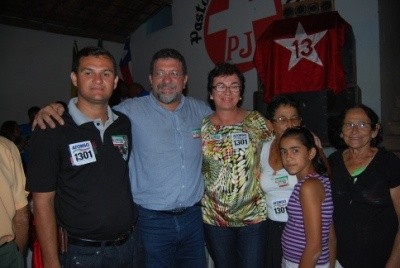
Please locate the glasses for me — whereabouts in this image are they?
[341,122,370,129]
[212,85,241,92]
[272,115,303,124]
[152,71,185,77]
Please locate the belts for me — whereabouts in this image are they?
[161,207,188,215]
[68,224,135,248]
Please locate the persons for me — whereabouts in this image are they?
[0,43,400,268]
